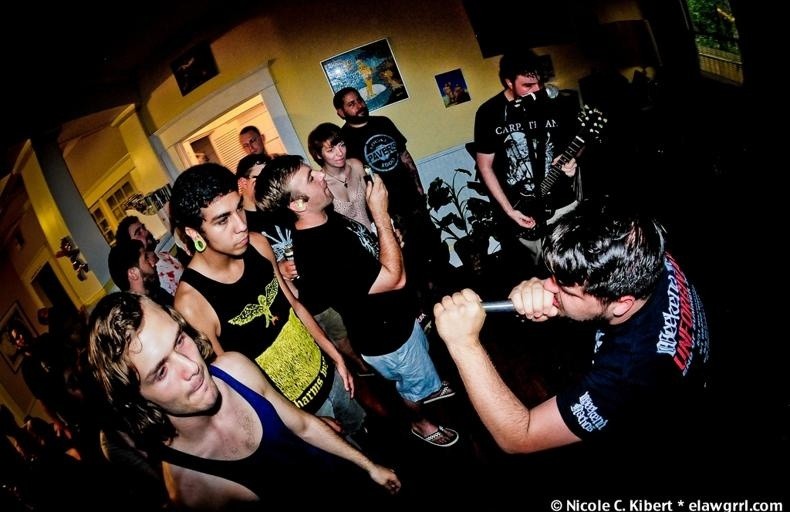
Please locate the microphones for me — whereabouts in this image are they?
[481,301,521,315]
[514,84,564,111]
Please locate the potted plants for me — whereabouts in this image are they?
[425,168,499,271]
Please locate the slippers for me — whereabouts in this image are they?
[423,381,459,405]
[410,425,459,448]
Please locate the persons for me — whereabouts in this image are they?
[433,197,712,453]
[117,215,188,295]
[168,163,376,457]
[237,126,271,175]
[308,123,372,232]
[333,87,451,294]
[255,155,460,448]
[236,155,273,232]
[474,47,577,230]
[0,317,85,512]
[86,293,401,512]
[108,239,215,367]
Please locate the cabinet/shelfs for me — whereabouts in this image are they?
[126,181,173,240]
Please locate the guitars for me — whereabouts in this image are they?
[505,104,608,240]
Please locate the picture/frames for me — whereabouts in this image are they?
[169,40,220,97]
[0,300,38,372]
[434,68,471,109]
[320,37,409,112]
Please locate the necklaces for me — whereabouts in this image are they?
[325,168,352,189]
